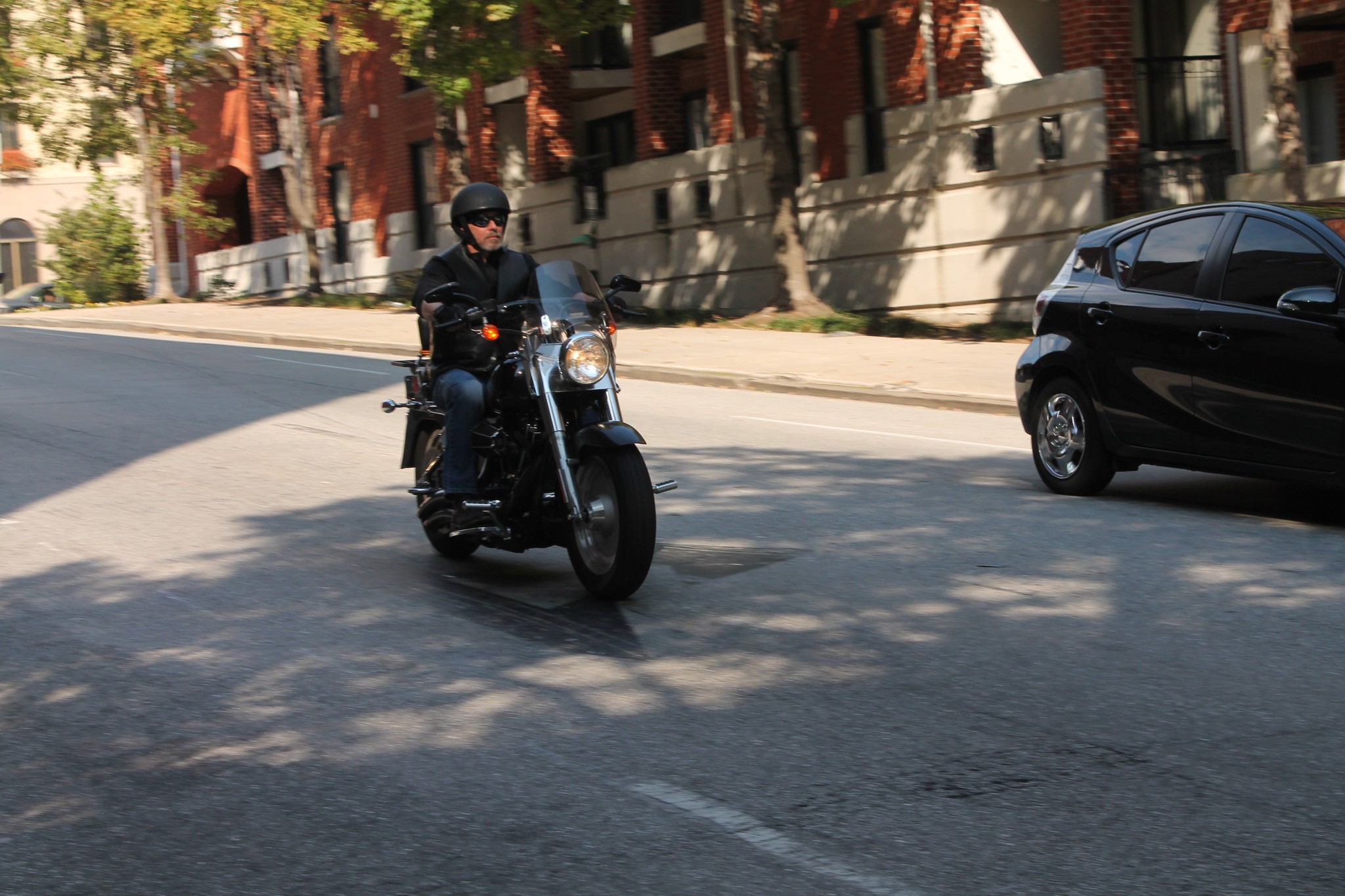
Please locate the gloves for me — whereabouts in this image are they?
[591,296,627,320]
[433,304,467,332]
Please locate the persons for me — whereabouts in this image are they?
[414,183,627,529]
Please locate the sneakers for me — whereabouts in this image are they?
[450,507,493,531]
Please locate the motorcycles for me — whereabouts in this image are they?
[381,258,678,601]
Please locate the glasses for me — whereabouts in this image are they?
[472,213,506,228]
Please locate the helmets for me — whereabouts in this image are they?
[450,182,512,220]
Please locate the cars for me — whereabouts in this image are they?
[1014,202,1345,497]
[0,282,71,315]
